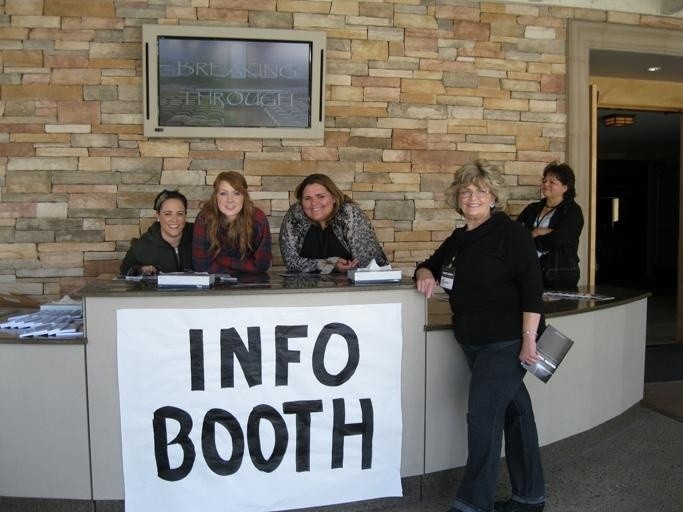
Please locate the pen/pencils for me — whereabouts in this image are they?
[117,276,142,279]
[215,277,238,281]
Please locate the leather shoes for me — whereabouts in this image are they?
[494,498,545,512]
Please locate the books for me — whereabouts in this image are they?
[522,324,574,384]
[0,309,84,338]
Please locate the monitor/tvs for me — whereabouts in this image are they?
[142,25,325,139]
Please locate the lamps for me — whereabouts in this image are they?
[602,113,637,127]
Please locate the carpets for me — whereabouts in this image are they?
[641,381,683,422]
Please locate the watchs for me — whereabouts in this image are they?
[522,330,538,336]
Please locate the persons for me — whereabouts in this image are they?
[516,162,585,288]
[119,189,193,276]
[279,173,389,274]
[412,160,545,512]
[194,171,273,274]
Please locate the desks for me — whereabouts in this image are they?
[0,306,93,501]
[77,271,426,501]
[425,286,652,474]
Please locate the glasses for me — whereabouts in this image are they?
[459,186,491,198]
